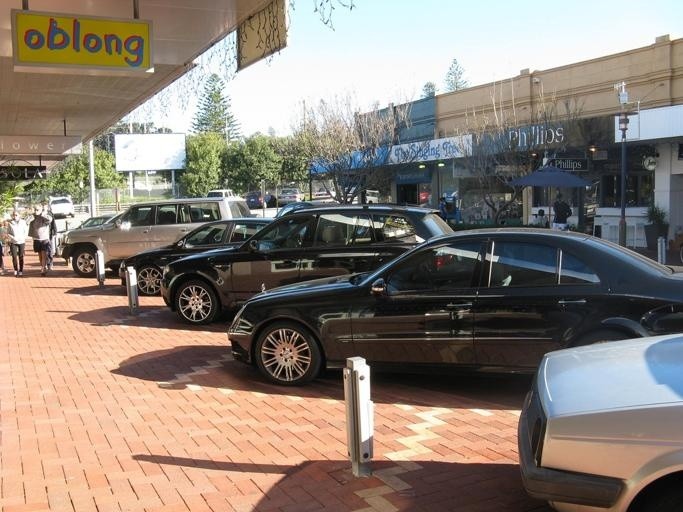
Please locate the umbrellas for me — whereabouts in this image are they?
[504,165,593,225]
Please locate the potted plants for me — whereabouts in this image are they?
[641,201,670,251]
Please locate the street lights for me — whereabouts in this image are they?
[619,83,664,247]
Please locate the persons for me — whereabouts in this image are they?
[0,203,57,277]
[531,209,548,228]
[552,194,572,230]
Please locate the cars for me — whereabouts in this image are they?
[246,190,276,209]
[278,186,305,207]
[118,201,327,296]
[227,226,683,386]
[315,186,392,204]
[518,333,683,512]
[462,194,522,225]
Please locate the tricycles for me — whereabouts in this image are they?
[439,191,461,225]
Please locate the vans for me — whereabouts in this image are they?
[208,189,235,197]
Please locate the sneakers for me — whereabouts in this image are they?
[14,271,23,277]
[0,269,9,276]
[39,266,53,276]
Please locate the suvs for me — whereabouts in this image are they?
[160,204,455,325]
[50,196,75,217]
[60,197,262,277]
